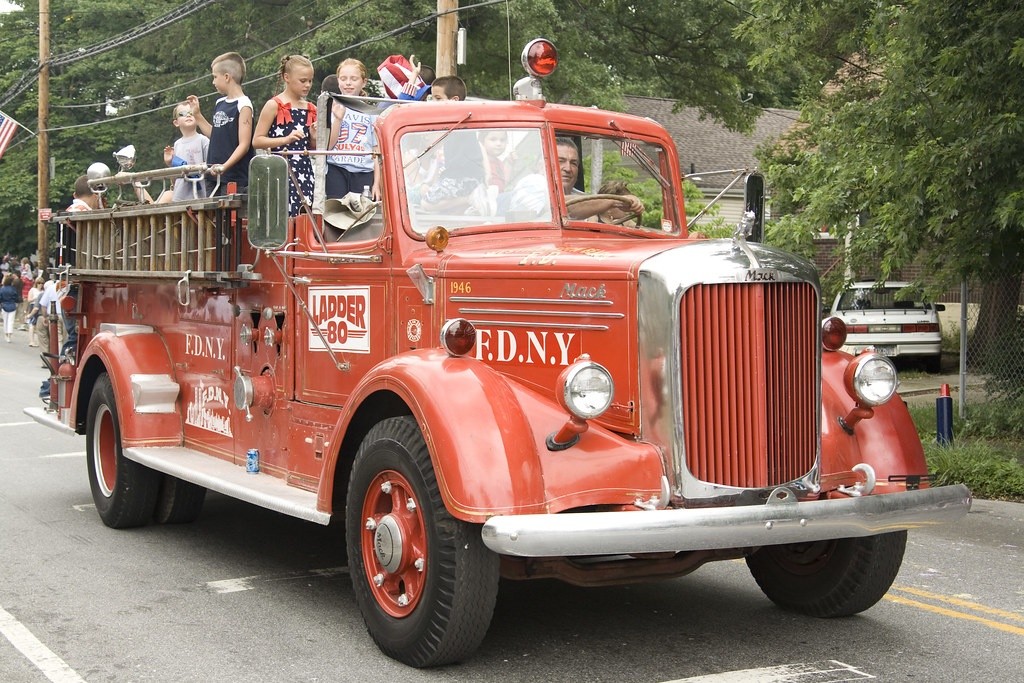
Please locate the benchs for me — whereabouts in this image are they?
[324,214,505,243]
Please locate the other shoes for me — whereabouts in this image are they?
[7,338,11,342]
[487,185,499,218]
[29,344,38,347]
[40,363,49,369]
[469,183,491,218]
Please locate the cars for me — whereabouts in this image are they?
[822,280,946,373]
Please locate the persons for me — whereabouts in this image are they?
[0,50,514,404]
[513,137,643,224]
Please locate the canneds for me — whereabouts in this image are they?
[246,449,259,474]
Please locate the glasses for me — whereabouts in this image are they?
[176,110,193,120]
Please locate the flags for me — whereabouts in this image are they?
[0,112,17,160]
[620,140,637,158]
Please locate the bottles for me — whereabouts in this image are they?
[362,185,372,202]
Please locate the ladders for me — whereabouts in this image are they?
[45,196,262,283]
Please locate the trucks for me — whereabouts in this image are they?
[23,37,975,673]
